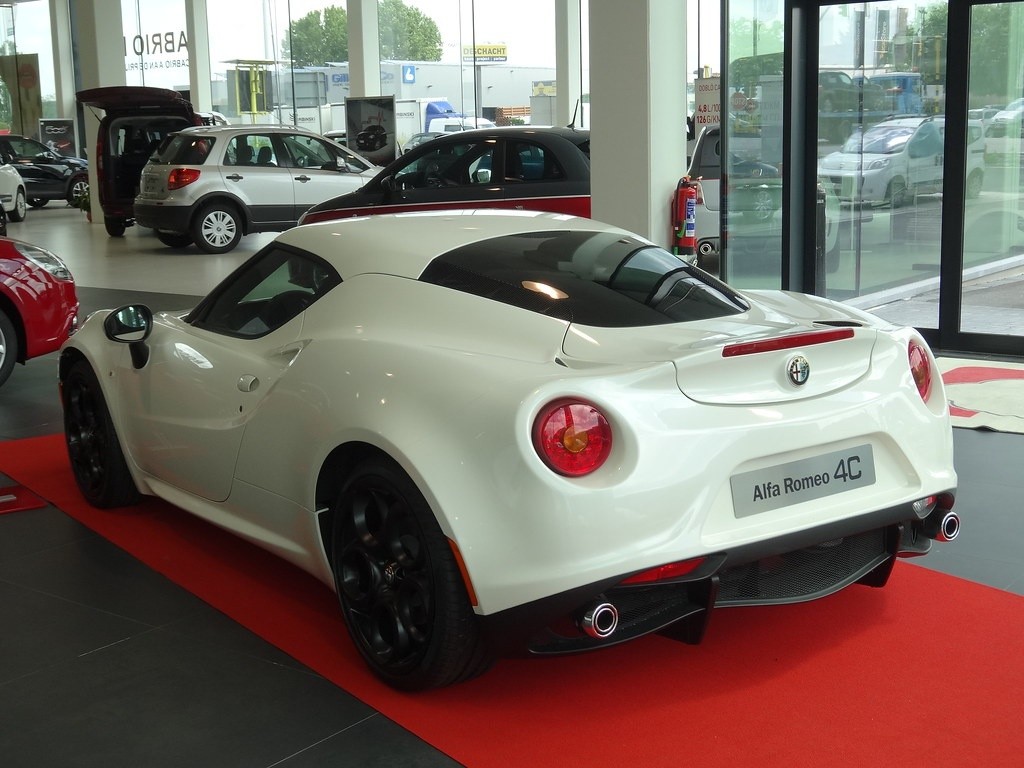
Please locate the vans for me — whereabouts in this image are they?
[816,117,986,209]
[428,117,498,135]
[75,86,218,236]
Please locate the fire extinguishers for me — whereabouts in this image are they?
[673,174,695,248]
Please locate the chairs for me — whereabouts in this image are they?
[506,152,524,182]
[255,146,275,166]
[433,155,469,185]
[235,146,254,166]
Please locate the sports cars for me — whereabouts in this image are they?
[58,208,962,694]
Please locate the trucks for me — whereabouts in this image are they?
[816,69,923,142]
[330,97,467,146]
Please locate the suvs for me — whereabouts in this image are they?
[684,124,841,275]
[132,123,405,255]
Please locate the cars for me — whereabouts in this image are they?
[0,135,89,210]
[323,130,404,161]
[401,131,451,155]
[292,122,591,223]
[0,233,80,387]
[356,125,387,151]
[819,71,862,112]
[967,105,1024,168]
[0,155,28,237]
